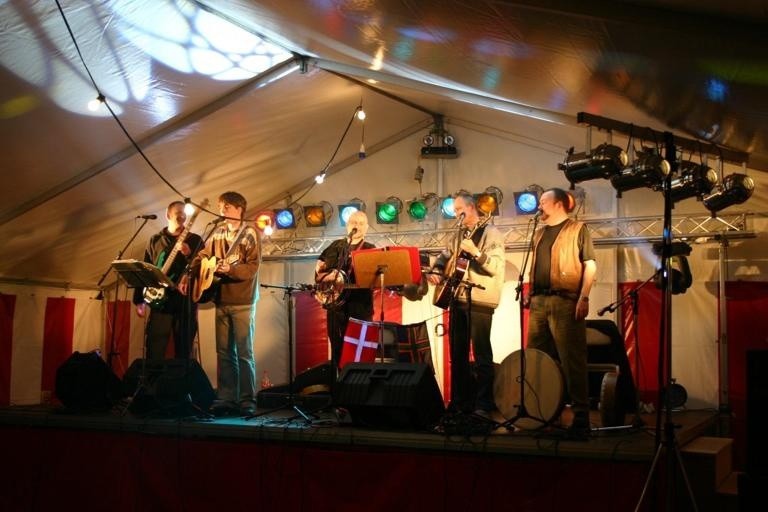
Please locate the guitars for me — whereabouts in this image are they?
[143,199,209,304]
[433,210,491,310]
[192,254,241,304]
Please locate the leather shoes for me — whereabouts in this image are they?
[238,405,257,415]
[208,403,237,417]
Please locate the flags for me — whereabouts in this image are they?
[393,320,435,375]
[338,317,380,368]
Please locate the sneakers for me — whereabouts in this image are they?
[567,412,592,441]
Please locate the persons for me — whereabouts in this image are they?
[315,211,376,365]
[133,201,205,359]
[177,192,262,416]
[526,188,597,432]
[429,193,505,412]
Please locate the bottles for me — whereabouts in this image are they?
[261,369,271,389]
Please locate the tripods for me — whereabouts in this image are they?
[494,223,566,433]
[243,291,314,424]
[631,193,700,512]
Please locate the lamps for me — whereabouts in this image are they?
[255,184,544,233]
[652,242,693,295]
[556,108,754,219]
[421,134,457,160]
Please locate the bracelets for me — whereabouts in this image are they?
[579,296,589,302]
[474,251,481,258]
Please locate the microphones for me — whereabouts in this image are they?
[529,207,544,220]
[206,215,226,225]
[455,211,467,227]
[137,215,158,220]
[348,228,357,237]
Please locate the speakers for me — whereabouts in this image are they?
[331,362,444,433]
[55,351,123,412]
[122,358,214,419]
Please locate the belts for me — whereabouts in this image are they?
[530,289,576,301]
